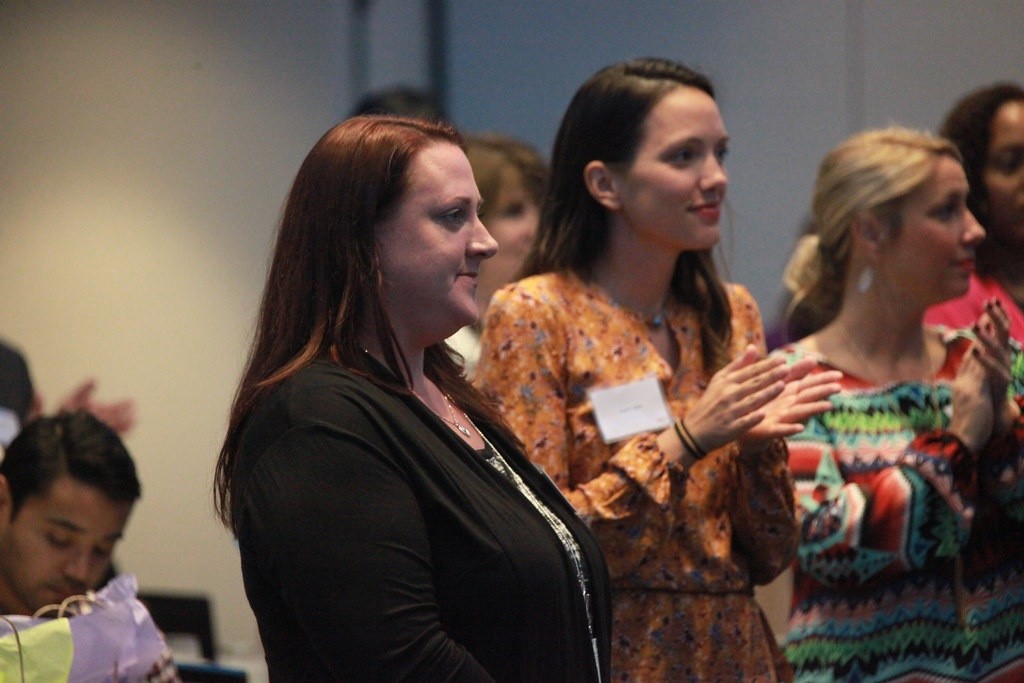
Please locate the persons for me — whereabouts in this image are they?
[766,83,1024,683]
[0,340,184,683]
[210,113,614,683]
[352,58,842,683]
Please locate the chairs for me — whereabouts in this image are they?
[137,588,246,683]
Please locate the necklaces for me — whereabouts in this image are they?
[627,301,673,330]
[421,394,471,437]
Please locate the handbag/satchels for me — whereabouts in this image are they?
[1,572,181,683]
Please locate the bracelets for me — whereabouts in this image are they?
[674,418,707,461]
